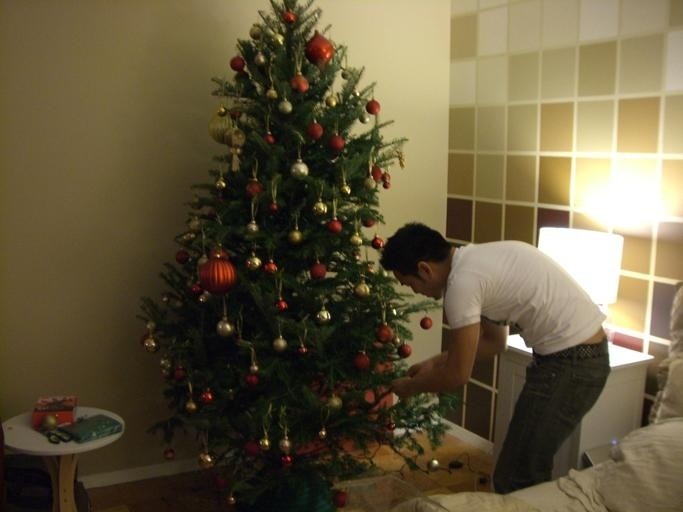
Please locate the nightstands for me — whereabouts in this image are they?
[493,334,654,469]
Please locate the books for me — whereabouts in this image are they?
[57,414,123,444]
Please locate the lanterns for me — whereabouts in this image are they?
[199,256,239,297]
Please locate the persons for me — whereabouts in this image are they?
[379,222,610,494]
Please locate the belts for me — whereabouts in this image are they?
[530,339,610,361]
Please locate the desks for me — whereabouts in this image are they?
[1,406,126,512]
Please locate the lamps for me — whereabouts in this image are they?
[537,226,625,315]
